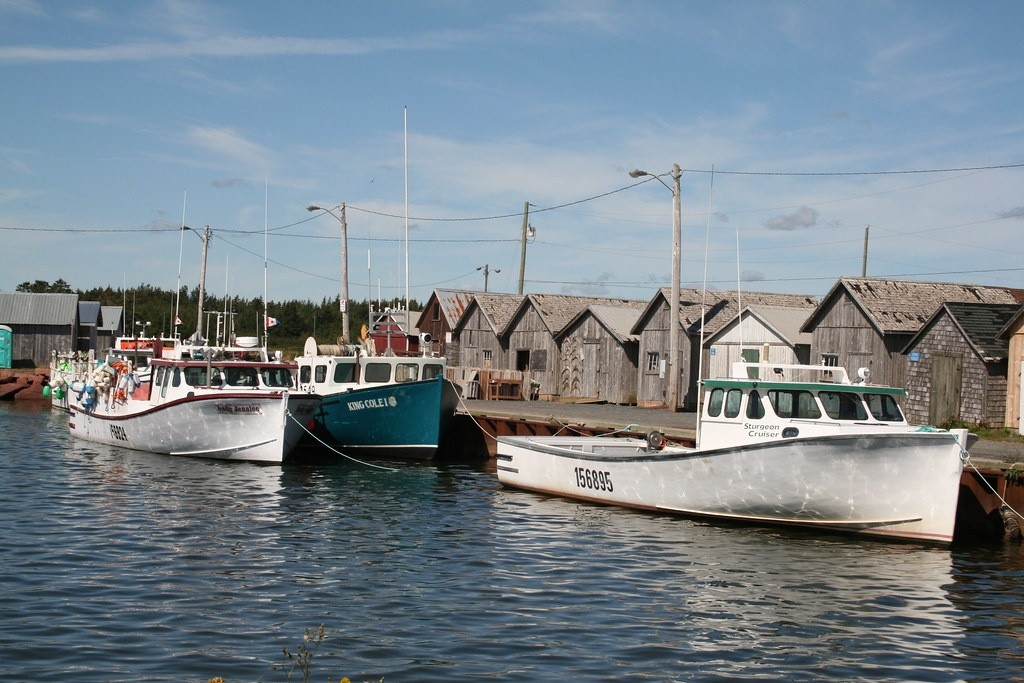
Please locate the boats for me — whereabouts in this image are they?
[70,174,323,467]
[50,272,183,413]
[290,105,463,462]
[497,164,979,547]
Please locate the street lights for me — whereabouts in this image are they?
[476,264,501,293]
[180,225,209,341]
[629,164,682,414]
[306,202,350,345]
